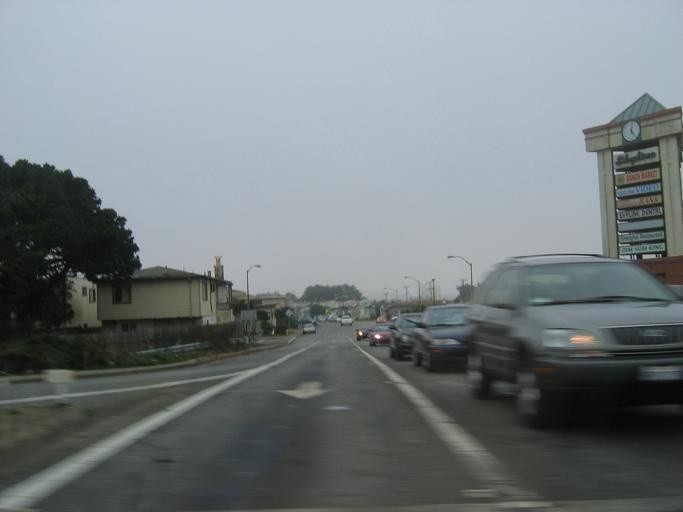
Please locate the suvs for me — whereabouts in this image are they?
[412,303,482,373]
[465,255,682,424]
[389,312,424,361]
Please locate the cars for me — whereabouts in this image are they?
[358,327,373,341]
[302,323,316,335]
[340,315,352,326]
[376,316,387,324]
[367,324,392,347]
[336,317,340,323]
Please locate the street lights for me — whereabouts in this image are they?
[384,288,399,315]
[245,264,261,344]
[404,276,421,312]
[447,255,473,305]
